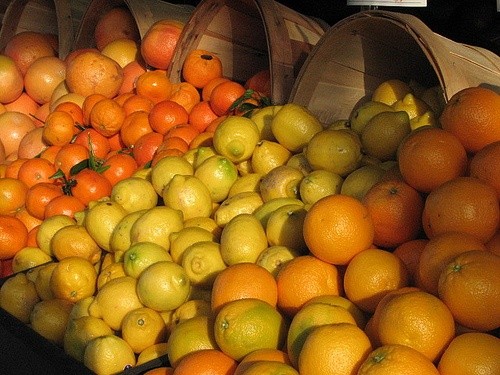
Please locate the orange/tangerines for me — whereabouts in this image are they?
[0,9,272,279]
[145,85,500,375]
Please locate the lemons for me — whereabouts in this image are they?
[0,78,441,375]
